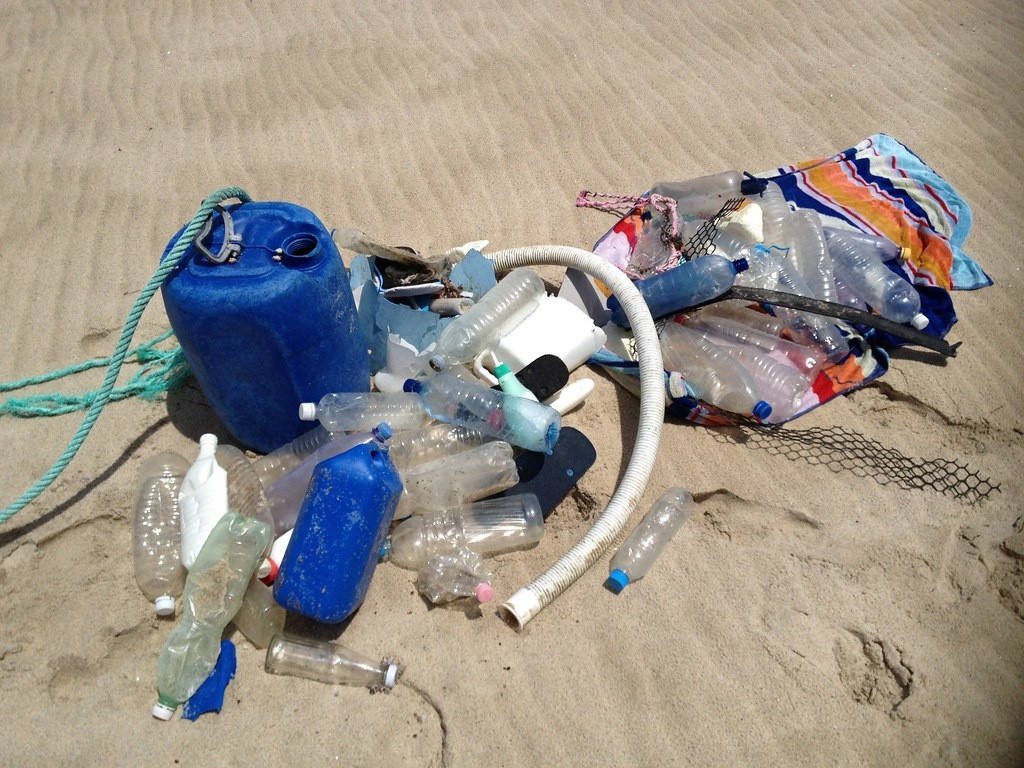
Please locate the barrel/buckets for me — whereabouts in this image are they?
[161,201,371,455]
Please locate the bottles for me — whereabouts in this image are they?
[606,486,694,591]
[607,169,929,422]
[132,267,561,722]
